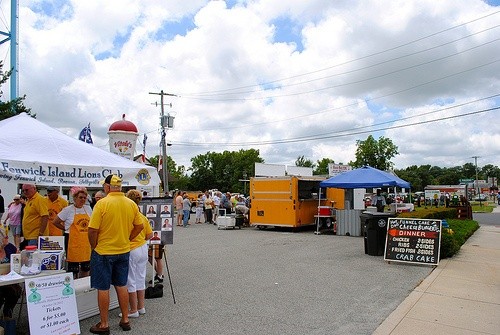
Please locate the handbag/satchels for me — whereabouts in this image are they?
[145,283,163,299]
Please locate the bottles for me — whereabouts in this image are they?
[384,206,388,213]
[365,198,371,206]
[20,245,42,276]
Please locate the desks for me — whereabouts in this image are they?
[0,270,66,324]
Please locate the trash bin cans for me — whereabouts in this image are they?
[359,211,397,255]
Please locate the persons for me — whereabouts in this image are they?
[372,189,386,213]
[0,190,4,219]
[0,238,22,322]
[89,189,105,210]
[165,190,250,228]
[88,174,144,335]
[139,205,171,241]
[21,180,51,251]
[417,194,449,208]
[497,192,500,204]
[148,244,165,283]
[4,194,30,252]
[119,189,153,318]
[44,186,69,235]
[52,186,95,279]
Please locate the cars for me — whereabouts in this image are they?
[475,193,487,201]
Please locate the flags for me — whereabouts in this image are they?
[157,157,163,172]
[79,127,93,144]
[142,140,145,162]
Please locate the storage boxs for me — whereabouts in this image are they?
[38,236,65,270]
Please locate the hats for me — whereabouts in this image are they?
[99,174,122,186]
[377,189,380,193]
[227,192,250,200]
[381,192,387,195]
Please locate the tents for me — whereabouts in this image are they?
[0,112,162,287]
[315,166,411,235]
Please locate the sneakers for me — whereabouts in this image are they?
[148,273,164,284]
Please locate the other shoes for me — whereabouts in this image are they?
[89,322,110,335]
[183,224,190,228]
[177,224,183,226]
[205,220,217,225]
[195,222,202,224]
[187,222,191,225]
[119,319,130,331]
[128,306,146,315]
[0,309,13,322]
[119,310,139,317]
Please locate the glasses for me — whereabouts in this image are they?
[47,190,56,193]
[22,189,28,193]
[79,196,86,199]
[14,199,19,201]
